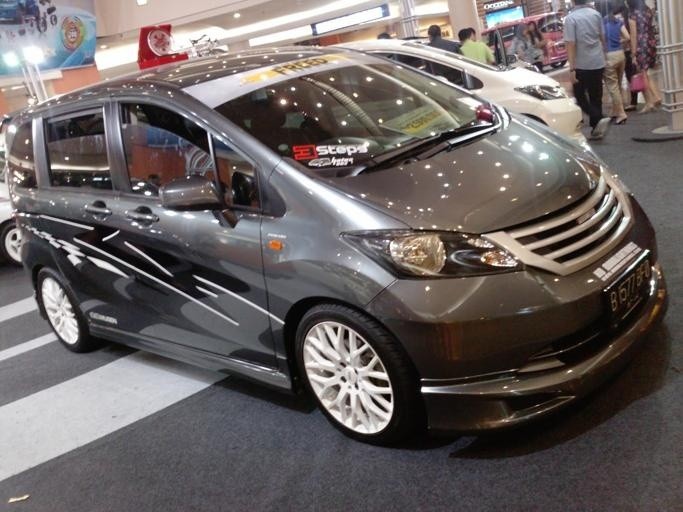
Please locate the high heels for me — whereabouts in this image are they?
[638,98,661,114]
[606,110,627,124]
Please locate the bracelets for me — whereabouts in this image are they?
[569,68,575,72]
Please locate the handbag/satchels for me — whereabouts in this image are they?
[629,71,646,93]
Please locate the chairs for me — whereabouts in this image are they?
[251,107,304,156]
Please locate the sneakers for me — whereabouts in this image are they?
[587,118,611,140]
[624,105,637,111]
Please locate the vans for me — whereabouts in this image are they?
[483,12,567,71]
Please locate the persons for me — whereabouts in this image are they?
[528,21,543,72]
[625,0,663,114]
[510,23,548,65]
[467,27,476,42]
[377,33,390,39]
[561,1,611,140]
[593,0,631,126]
[606,0,638,113]
[427,25,464,55]
[458,29,495,66]
